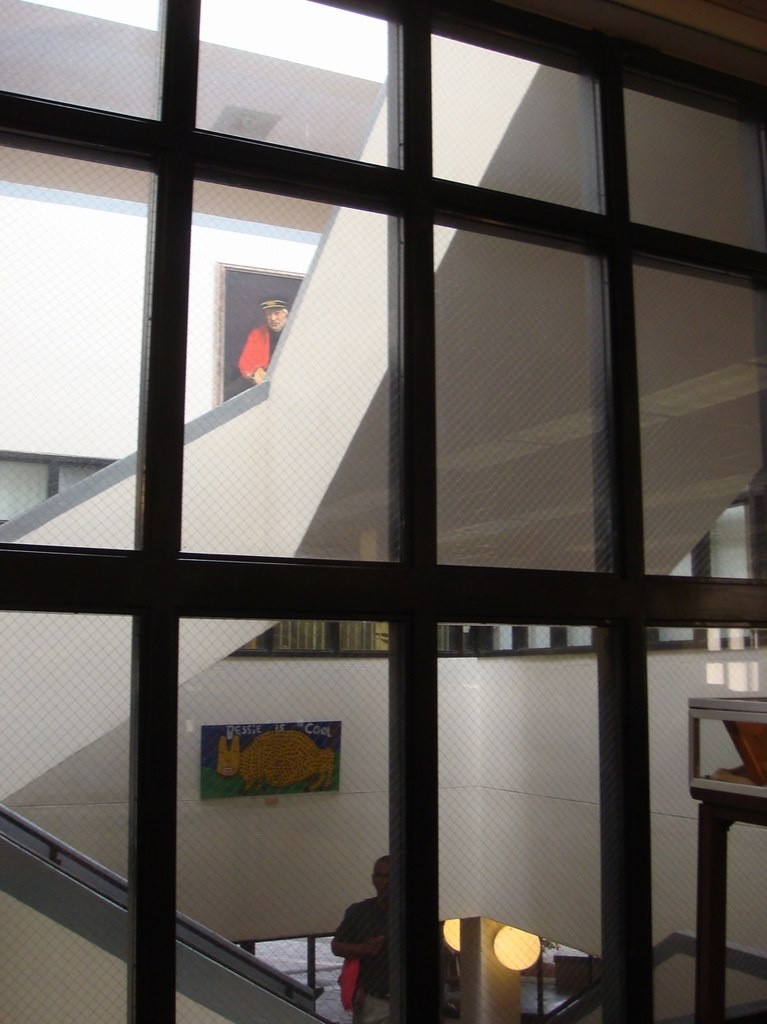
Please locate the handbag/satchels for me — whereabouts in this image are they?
[338,956,365,1012]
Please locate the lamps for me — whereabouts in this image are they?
[492,925,542,970]
[441,918,460,951]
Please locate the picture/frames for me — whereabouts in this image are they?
[214,258,310,411]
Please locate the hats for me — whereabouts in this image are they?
[257,296,288,309]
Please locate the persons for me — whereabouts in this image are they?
[331,856,391,1024]
[239,298,289,385]
